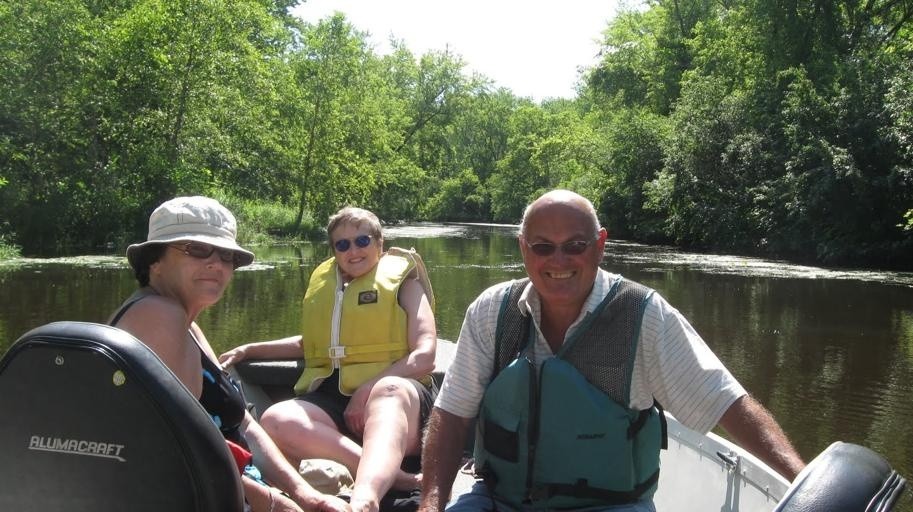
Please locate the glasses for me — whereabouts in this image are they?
[525,234,597,256]
[335,235,376,251]
[164,242,234,262]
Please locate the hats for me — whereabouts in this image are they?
[126,195,255,271]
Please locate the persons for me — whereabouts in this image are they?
[103,195,352,512]
[416,188,806,512]
[218,206,442,512]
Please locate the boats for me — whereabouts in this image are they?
[0,321,790,512]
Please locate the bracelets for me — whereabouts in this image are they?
[268,490,275,512]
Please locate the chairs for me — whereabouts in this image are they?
[1,321,244,511]
